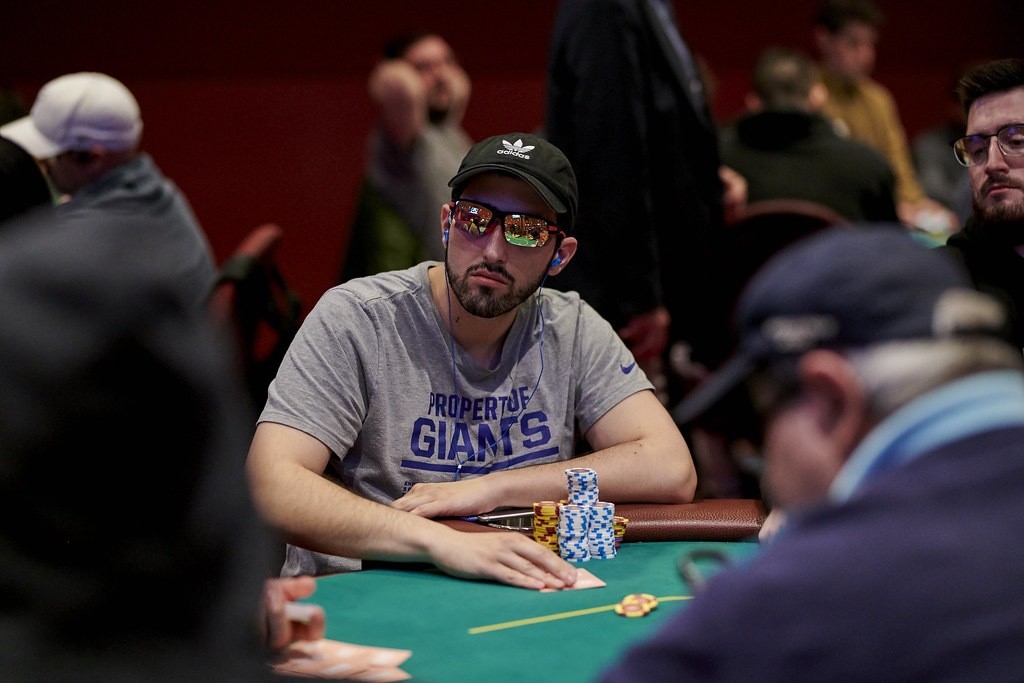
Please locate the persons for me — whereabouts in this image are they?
[546,0,749,494]
[812,0,958,232]
[452,213,487,235]
[719,51,900,230]
[597,228,1024,682]
[336,33,473,281]
[0,70,326,683]
[946,60,1024,356]
[512,226,540,240]
[245,132,697,590]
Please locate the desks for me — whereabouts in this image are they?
[267,492,771,682]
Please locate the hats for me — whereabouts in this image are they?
[447,132,579,239]
[0,72,143,161]
[675,228,1024,447]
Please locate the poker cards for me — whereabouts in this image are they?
[539,566,607,593]
[264,637,413,683]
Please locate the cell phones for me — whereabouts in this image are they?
[478,504,536,521]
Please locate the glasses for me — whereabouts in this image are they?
[450,197,566,248]
[950,122,1024,167]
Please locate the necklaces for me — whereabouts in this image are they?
[445,273,544,481]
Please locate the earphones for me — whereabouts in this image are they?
[550,255,565,267]
[443,228,450,245]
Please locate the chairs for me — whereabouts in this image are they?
[192,198,874,500]
